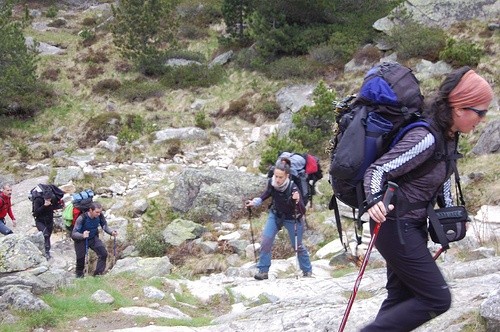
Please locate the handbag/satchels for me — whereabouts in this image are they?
[428,205,468,244]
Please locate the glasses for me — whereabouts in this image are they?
[461,107,488,117]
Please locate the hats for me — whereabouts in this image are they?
[41,190,55,199]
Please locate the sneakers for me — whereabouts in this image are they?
[299,270,312,279]
[253,272,268,280]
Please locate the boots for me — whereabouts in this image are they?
[44,237,51,261]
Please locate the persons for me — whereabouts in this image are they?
[357,66,494,332]
[0,182,17,235]
[244,156,312,281]
[32,185,66,260]
[71,201,119,279]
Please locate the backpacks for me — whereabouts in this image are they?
[62,197,100,242]
[328,61,446,212]
[268,150,324,220]
[28,183,65,205]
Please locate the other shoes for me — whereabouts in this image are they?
[93,271,103,276]
